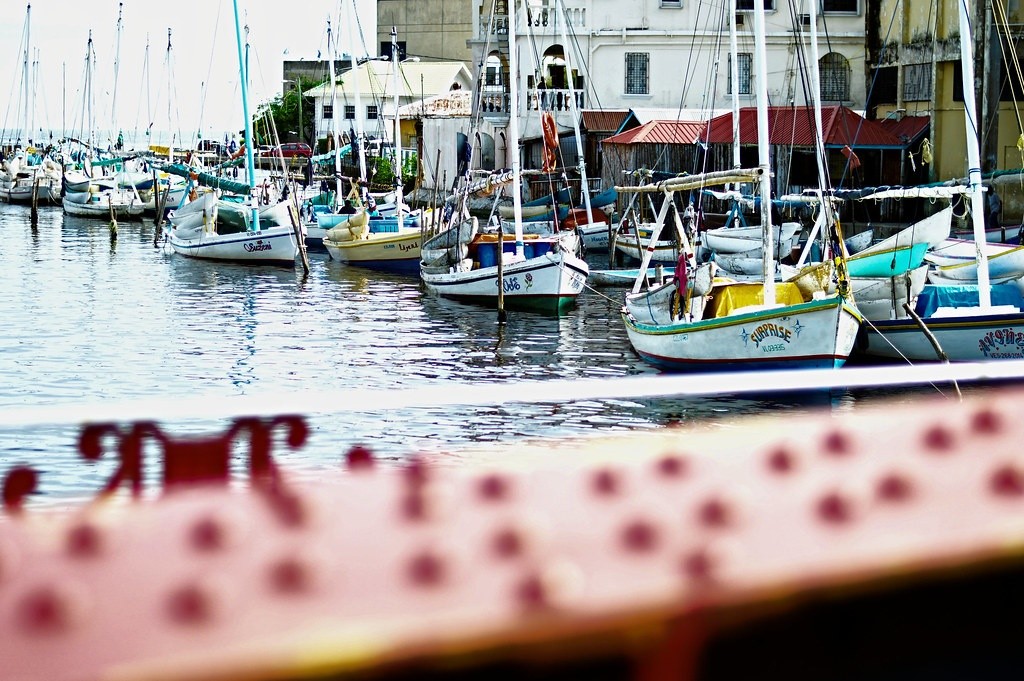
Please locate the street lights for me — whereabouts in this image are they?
[281,79,303,142]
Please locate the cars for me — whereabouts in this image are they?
[261,143,312,158]
[253,145,275,156]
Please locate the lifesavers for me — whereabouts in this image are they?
[542,112,559,149]
[542,152,558,173]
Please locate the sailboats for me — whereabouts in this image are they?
[1,1,1024,370]
